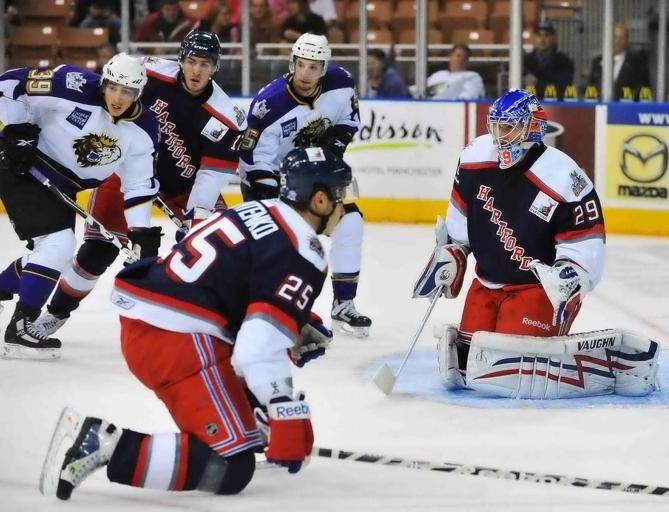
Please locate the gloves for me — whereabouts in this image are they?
[122,226,164,268]
[262,392,317,474]
[2,123,41,178]
[174,207,214,244]
[286,312,335,368]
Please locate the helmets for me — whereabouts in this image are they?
[279,146,354,204]
[177,30,221,69]
[288,32,332,78]
[97,52,148,104]
[486,88,548,170]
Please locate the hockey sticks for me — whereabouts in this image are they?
[373,271,449,396]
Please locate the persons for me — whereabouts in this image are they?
[589,26,651,102]
[356,49,411,101]
[33,32,249,338]
[411,90,660,398]
[522,24,575,99]
[79,1,120,47]
[238,33,374,329]
[55,147,353,503]
[407,43,485,101]
[131,0,328,55]
[0,52,157,349]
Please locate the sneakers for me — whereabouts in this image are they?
[37,406,124,502]
[31,309,69,337]
[329,297,373,340]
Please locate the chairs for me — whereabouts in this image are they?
[316,0,576,69]
[176,1,205,19]
[0,0,111,75]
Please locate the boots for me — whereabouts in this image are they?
[1,302,63,362]
